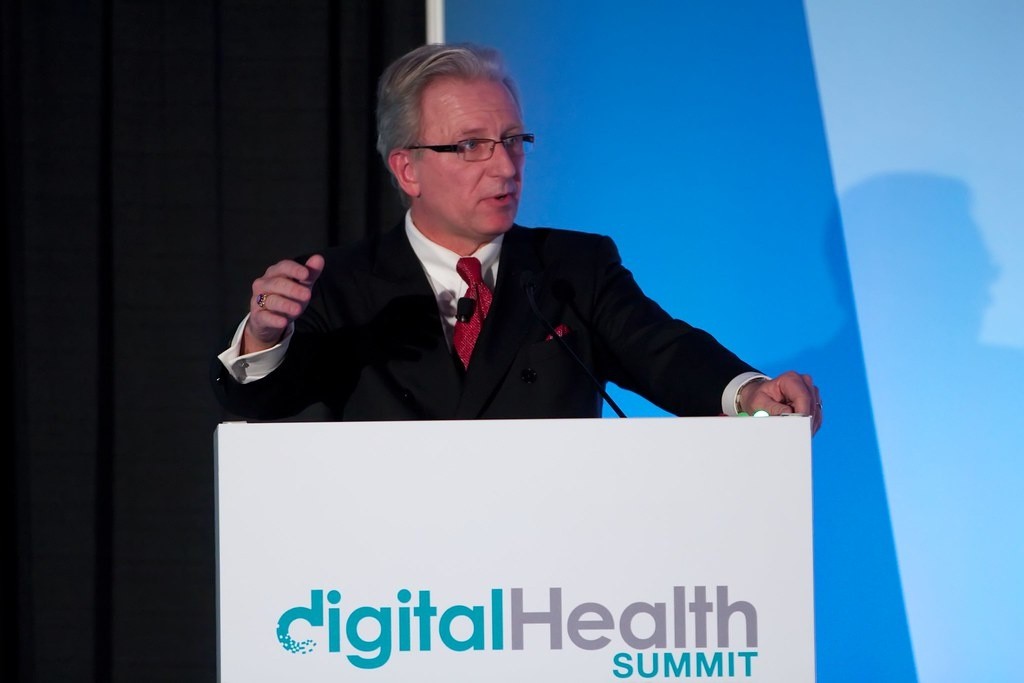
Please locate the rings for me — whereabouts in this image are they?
[257,293,273,310]
[816,400,822,410]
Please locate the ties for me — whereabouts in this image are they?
[453,257,493,370]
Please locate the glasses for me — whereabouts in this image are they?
[404,133,535,162]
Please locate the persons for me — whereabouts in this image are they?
[210,44,822,439]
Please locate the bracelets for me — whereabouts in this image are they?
[735,379,765,412]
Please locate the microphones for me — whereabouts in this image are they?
[521,270,628,418]
